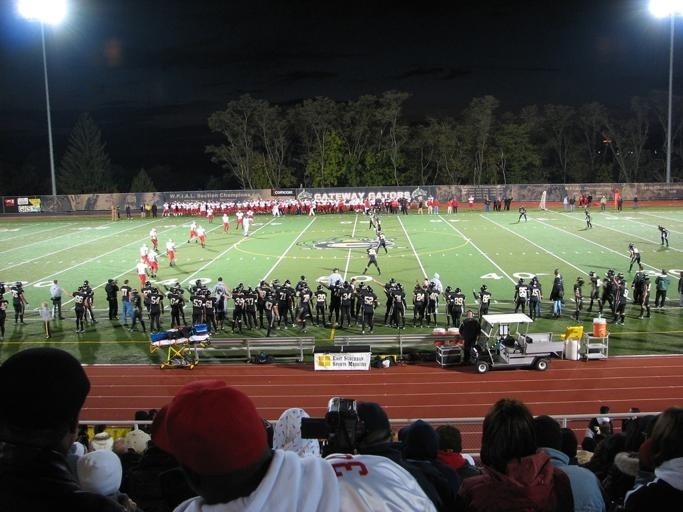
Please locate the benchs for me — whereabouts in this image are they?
[334,334,461,358]
[184,336,316,363]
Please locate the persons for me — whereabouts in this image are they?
[362,228,389,276]
[1,347,682,512]
[110,194,639,229]
[1,268,682,338]
[166,239,176,266]
[137,243,158,288]
[188,221,208,248]
[150,228,158,251]
[658,224,670,247]
[627,242,644,272]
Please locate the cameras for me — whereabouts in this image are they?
[301,398,362,445]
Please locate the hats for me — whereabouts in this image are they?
[77,450,123,496]
[1,347,91,446]
[94,432,115,451]
[399,418,437,457]
[149,379,268,472]
[124,430,148,452]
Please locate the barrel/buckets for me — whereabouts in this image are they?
[448,328,464,344]
[432,328,445,346]
[593,318,606,337]
[566,339,581,361]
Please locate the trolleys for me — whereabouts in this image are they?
[150,339,211,370]
